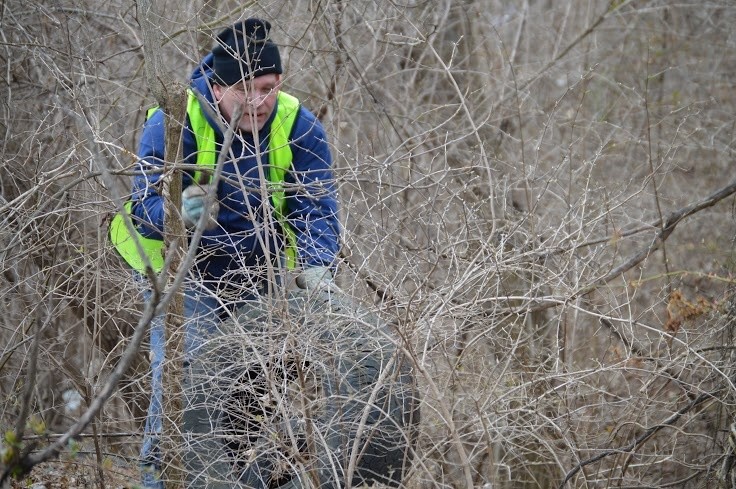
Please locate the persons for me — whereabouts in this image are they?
[108,17,348,489]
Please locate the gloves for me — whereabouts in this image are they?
[182,185,218,230]
[297,266,343,293]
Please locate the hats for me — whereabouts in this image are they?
[212,19,283,83]
